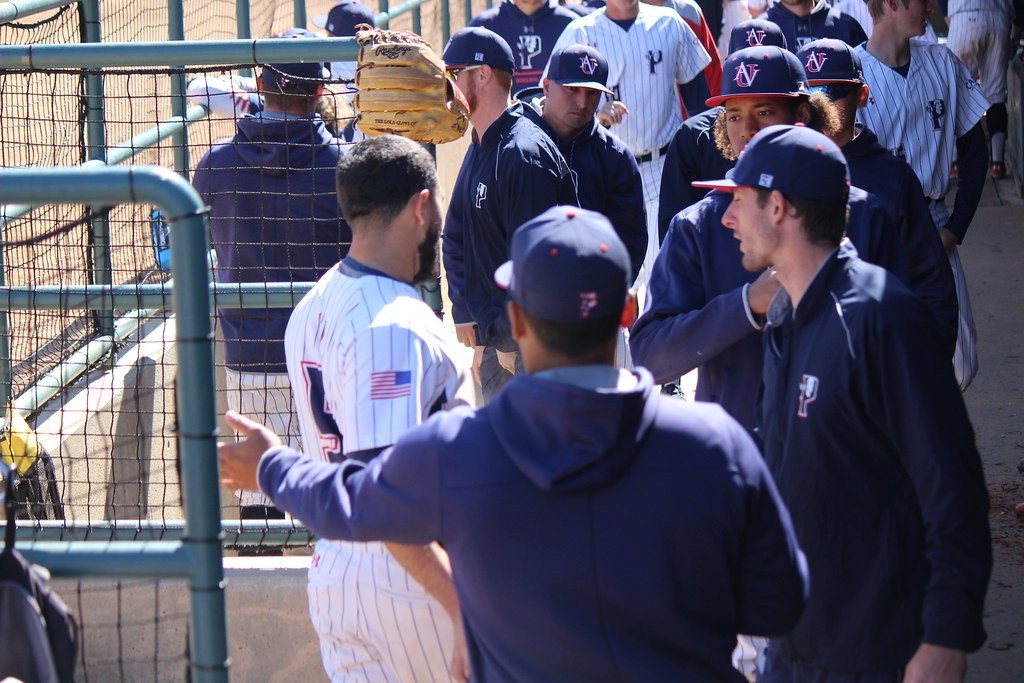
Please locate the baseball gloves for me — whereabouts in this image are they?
[354,23,470,145]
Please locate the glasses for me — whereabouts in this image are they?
[446,62,485,81]
[807,84,856,103]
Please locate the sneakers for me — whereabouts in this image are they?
[950,165,959,178]
[992,163,1006,178]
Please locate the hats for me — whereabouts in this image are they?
[795,36,867,86]
[724,20,786,60]
[442,25,517,78]
[704,46,812,107]
[547,41,616,97]
[691,122,851,205]
[262,33,330,88]
[312,2,375,37]
[493,205,631,322]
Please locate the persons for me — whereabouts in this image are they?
[690,121,996,682]
[182,0,1021,558]
[215,200,811,683]
[628,43,957,464]
[283,135,484,683]
